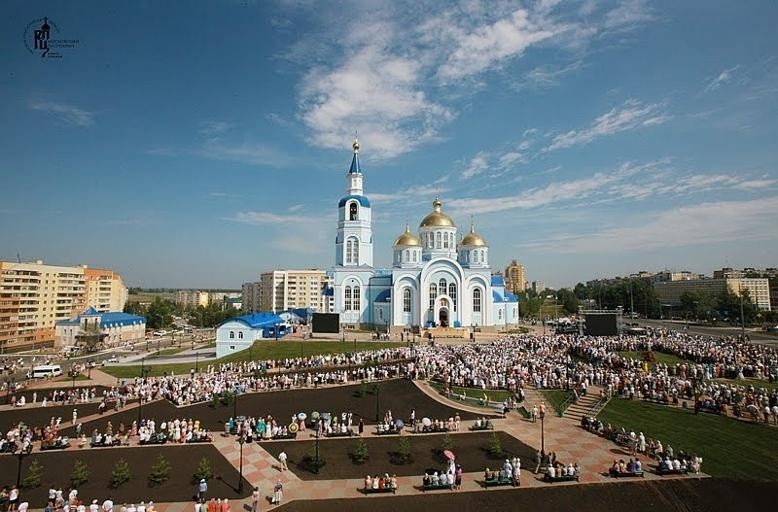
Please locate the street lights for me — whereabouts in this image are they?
[540,409,545,461]
[5,380,11,403]
[314,418,320,475]
[15,442,26,489]
[237,424,246,495]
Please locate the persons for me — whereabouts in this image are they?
[612,457,642,477]
[484,457,521,487]
[2,330,135,407]
[655,450,703,474]
[7,416,69,452]
[272,482,283,505]
[278,449,288,472]
[252,487,259,511]
[523,316,582,327]
[535,449,579,481]
[422,458,462,491]
[581,414,673,459]
[223,411,495,443]
[72,408,212,448]
[195,478,231,511]
[97,325,777,425]
[364,474,399,491]
[2,484,155,511]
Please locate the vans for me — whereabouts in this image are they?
[25,365,63,379]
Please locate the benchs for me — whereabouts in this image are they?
[486,478,513,485]
[364,488,397,495]
[545,475,580,482]
[423,484,452,490]
[610,470,645,478]
